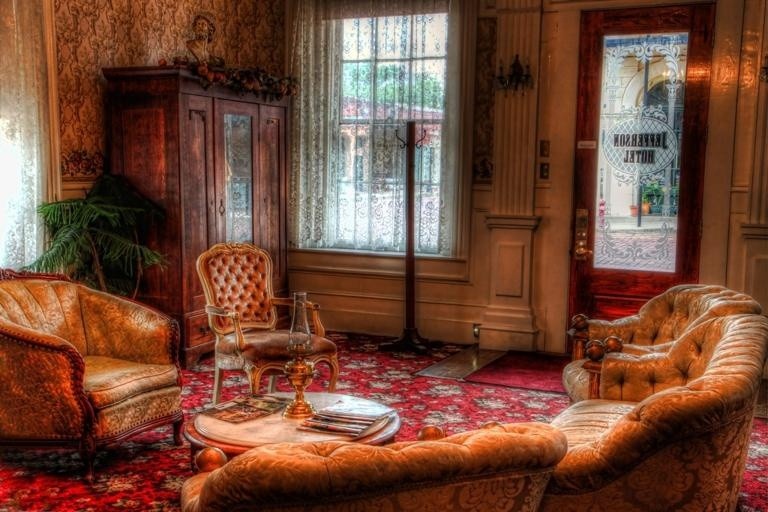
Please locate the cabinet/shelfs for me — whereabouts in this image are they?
[103,68,295,368]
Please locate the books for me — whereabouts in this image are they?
[296,393,399,443]
[230,388,291,415]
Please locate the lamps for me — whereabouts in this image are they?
[496,52,535,98]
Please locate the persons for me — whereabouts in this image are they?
[186,11,221,63]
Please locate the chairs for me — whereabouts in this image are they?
[0,264,185,471]
[194,242,341,405]
